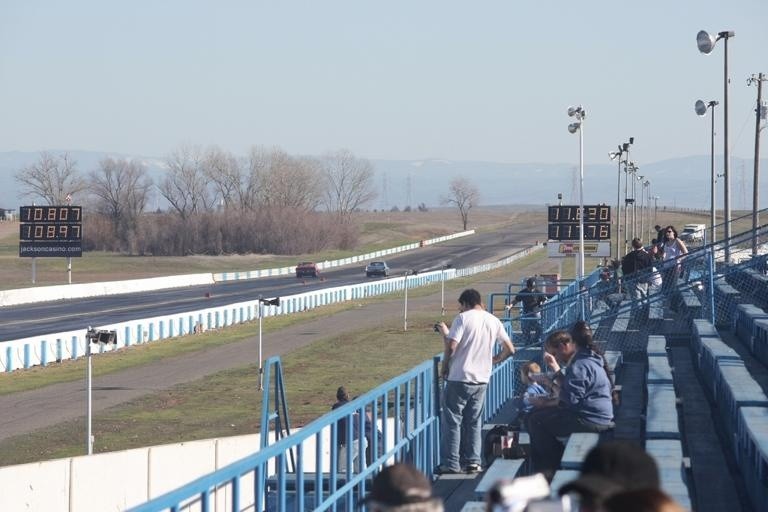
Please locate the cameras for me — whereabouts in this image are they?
[434,321,441,332]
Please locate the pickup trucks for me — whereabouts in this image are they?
[365,261,390,276]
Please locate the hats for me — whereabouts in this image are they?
[558,440,658,506]
[356,465,433,506]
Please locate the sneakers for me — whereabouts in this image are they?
[434,463,483,473]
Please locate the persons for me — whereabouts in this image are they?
[435,289,516,475]
[521,321,614,483]
[597,224,689,308]
[332,386,384,473]
[505,278,547,346]
[489,439,683,511]
[361,464,443,511]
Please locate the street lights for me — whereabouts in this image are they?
[746,74,767,256]
[696,27,735,266]
[257,295,280,391]
[82,325,117,455]
[566,104,586,279]
[404,269,415,332]
[441,264,453,314]
[690,98,720,251]
[608,136,661,270]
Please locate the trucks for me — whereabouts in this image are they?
[681,223,704,243]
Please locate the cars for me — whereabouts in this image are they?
[295,261,320,278]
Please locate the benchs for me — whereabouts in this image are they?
[461,253,768,512]
